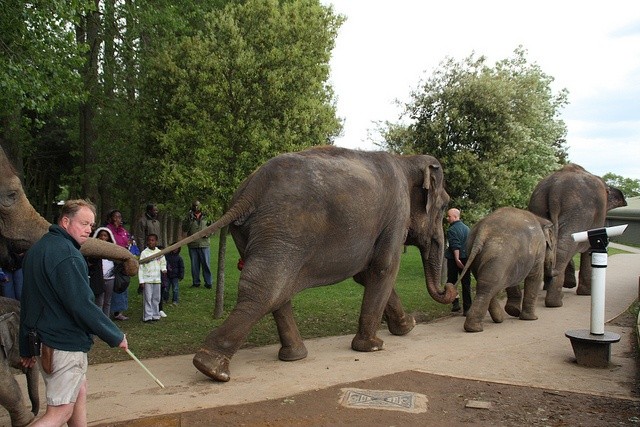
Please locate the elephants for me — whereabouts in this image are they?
[103,145,456,380]
[0,146,139,277]
[528,164,628,308]
[0,296,46,427]
[436,206,553,332]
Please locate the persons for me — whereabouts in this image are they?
[1,270,23,302]
[93,227,117,318]
[182,200,213,290]
[105,209,133,321]
[18,197,129,427]
[162,242,185,306]
[130,239,140,256]
[136,203,164,294]
[443,207,472,317]
[137,234,166,325]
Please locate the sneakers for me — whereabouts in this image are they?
[112,314,128,320]
[160,311,167,318]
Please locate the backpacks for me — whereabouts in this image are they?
[112,262,130,294]
